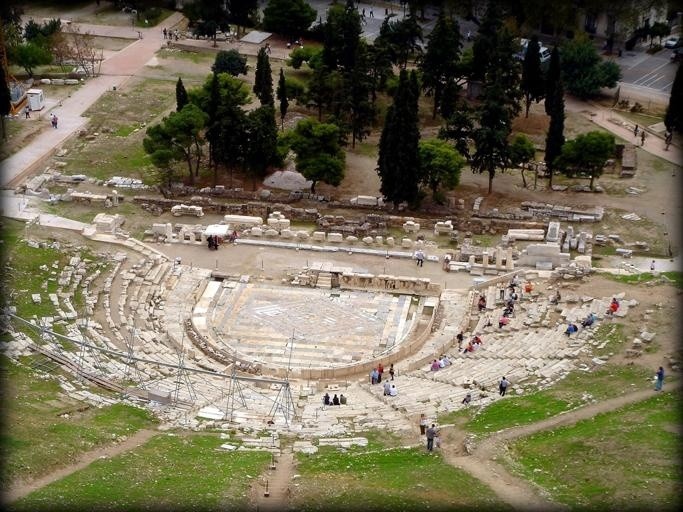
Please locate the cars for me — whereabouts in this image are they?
[664,35,680,48]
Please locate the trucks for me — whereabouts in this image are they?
[511,37,551,64]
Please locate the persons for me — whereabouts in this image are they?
[526,281,533,292]
[431,355,451,371]
[653,365,665,391]
[161,25,304,60]
[650,259,656,270]
[499,376,508,397]
[49,113,54,128]
[51,115,58,130]
[462,393,472,405]
[24,105,30,120]
[419,413,436,452]
[499,274,520,327]
[212,235,221,249]
[324,393,347,405]
[383,380,397,396]
[206,235,213,249]
[606,298,618,315]
[371,363,395,384]
[442,256,450,272]
[564,324,578,336]
[415,247,425,268]
[229,229,239,243]
[458,331,481,353]
[582,313,595,328]
[478,296,486,311]
[555,291,561,304]
[483,315,493,328]
